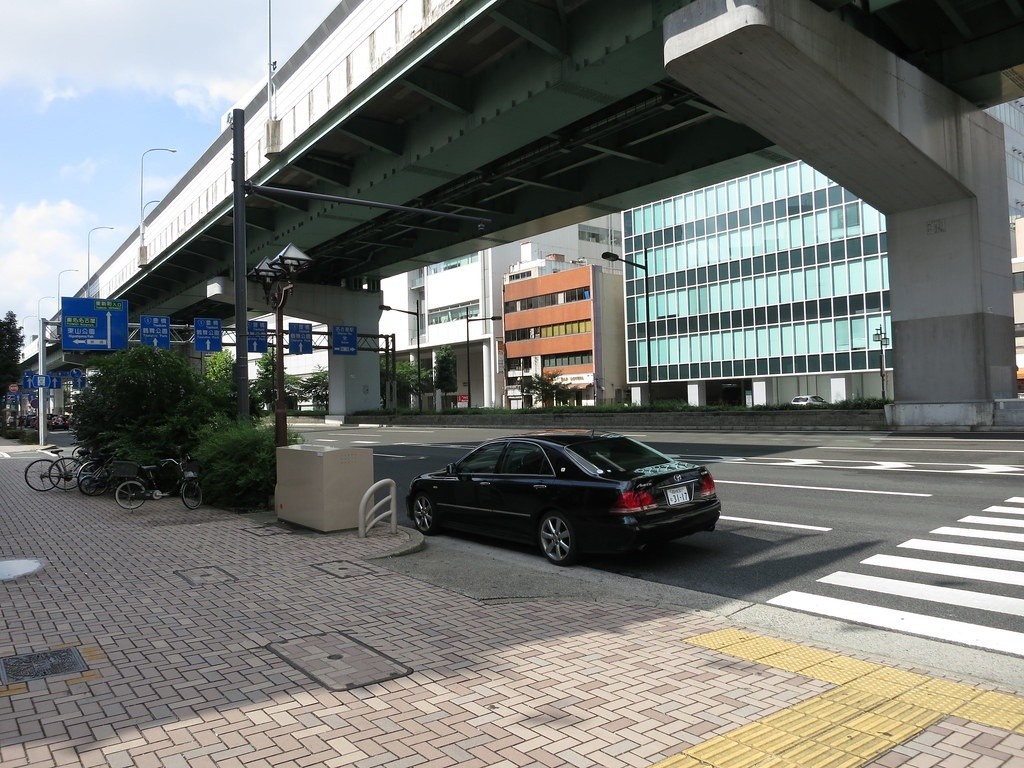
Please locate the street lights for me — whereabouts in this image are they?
[599,247,654,399]
[139,147,178,246]
[378,300,423,414]
[465,306,503,407]
[57,269,80,321]
[873,323,890,402]
[38,295,55,320]
[246,242,312,448]
[22,315,38,327]
[88,226,115,297]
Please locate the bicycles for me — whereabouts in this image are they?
[115,454,203,514]
[24,442,124,497]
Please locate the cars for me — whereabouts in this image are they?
[406,429,722,568]
[7,414,69,431]
[791,395,826,406]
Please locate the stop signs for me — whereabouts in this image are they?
[9,384,19,393]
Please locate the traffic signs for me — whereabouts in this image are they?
[61,297,130,351]
[140,314,170,351]
[288,322,313,355]
[248,320,268,353]
[333,325,357,355]
[194,317,222,352]
